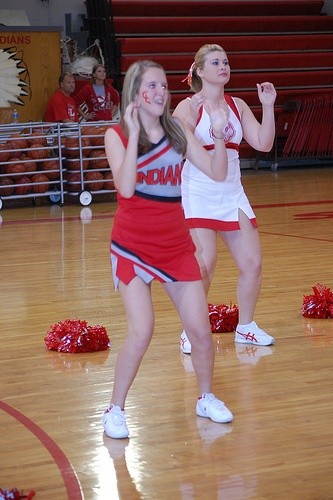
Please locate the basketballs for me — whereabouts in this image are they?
[0,120,118,197]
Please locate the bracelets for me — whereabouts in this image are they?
[212,133,225,140]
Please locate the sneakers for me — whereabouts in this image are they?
[102,403,130,439]
[234,321,275,346]
[180,329,192,354]
[195,392,234,424]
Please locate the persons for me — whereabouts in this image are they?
[44,71,79,123]
[102,61,230,438]
[172,43,277,354]
[74,64,119,121]
